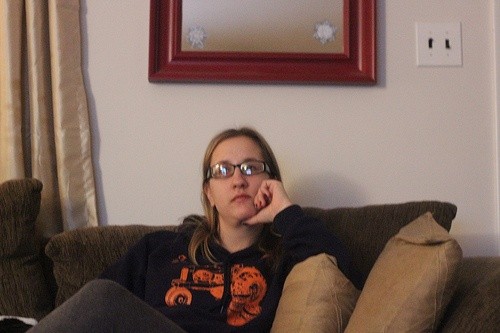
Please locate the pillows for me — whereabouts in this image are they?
[270,252,360,333]
[342,211,463,333]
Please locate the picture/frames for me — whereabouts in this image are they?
[148,0,377,86]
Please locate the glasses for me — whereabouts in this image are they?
[205,160,272,181]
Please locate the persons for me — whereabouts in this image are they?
[26,128,350,333]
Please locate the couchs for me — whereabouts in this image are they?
[0,177,500,333]
